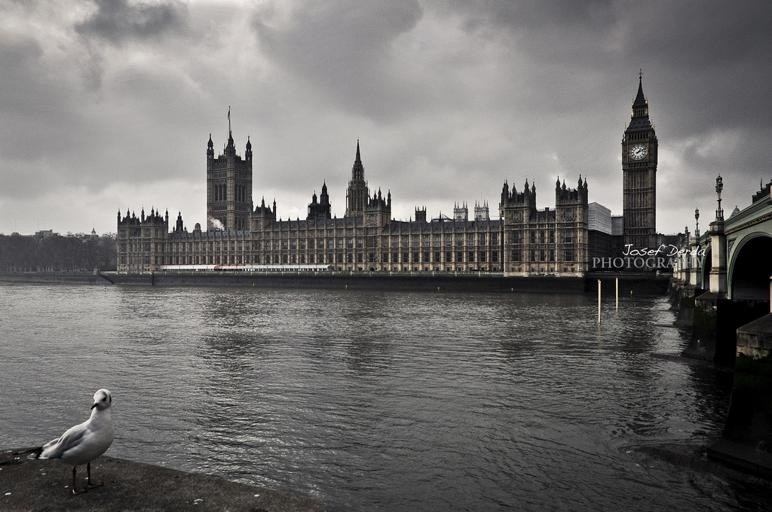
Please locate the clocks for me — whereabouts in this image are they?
[628,143,648,160]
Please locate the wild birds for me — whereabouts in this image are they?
[39,388,114,496]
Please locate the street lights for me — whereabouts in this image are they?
[715,171,725,222]
[684,226,689,247]
[694,207,700,243]
